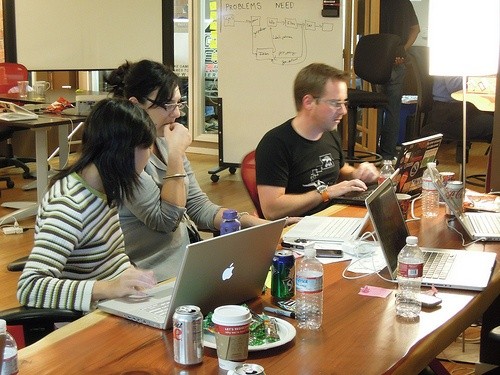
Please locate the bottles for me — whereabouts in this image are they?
[33,80,47,102]
[18,81,29,97]
[421,162,441,217]
[220,210,242,236]
[378,160,398,193]
[295,248,326,330]
[0,319,20,375]
[395,236,423,318]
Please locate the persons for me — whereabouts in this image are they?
[105,60,304,283]
[255,63,380,220]
[378,0,420,160]
[431,76,493,144]
[17,98,158,312]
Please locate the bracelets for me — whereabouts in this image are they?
[238,211,250,216]
[162,173,188,179]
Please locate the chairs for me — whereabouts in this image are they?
[0,63,51,178]
[347,34,401,167]
[406,45,488,181]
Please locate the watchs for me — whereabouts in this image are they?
[316,184,330,203]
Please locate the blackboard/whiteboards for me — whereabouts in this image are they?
[217,0,345,168]
[3,0,176,73]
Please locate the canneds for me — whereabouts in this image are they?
[270,249,296,300]
[172,305,203,365]
[445,181,463,215]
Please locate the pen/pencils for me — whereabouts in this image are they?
[280,314,281,315]
[281,242,304,249]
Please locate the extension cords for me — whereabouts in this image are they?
[2,225,23,234]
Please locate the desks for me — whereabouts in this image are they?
[0,89,113,224]
[11,174,500,375]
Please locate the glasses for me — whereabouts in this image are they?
[138,94,185,110]
[312,96,349,110]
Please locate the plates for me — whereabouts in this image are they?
[205,313,298,352]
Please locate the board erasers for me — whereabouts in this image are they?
[321,9,337,17]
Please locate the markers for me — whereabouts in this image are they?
[264,306,295,317]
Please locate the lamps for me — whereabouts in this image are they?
[428,1,499,192]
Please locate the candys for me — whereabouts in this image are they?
[202,304,280,345]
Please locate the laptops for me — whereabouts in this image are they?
[432,178,499,240]
[98,218,286,330]
[365,178,500,291]
[283,168,400,245]
[332,133,443,203]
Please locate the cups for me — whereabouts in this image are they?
[438,171,456,205]
[396,193,412,220]
[211,305,252,371]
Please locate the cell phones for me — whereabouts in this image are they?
[394,290,442,308]
[316,249,343,258]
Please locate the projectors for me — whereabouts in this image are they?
[76,95,115,115]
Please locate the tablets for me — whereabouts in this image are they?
[0,112,36,122]
